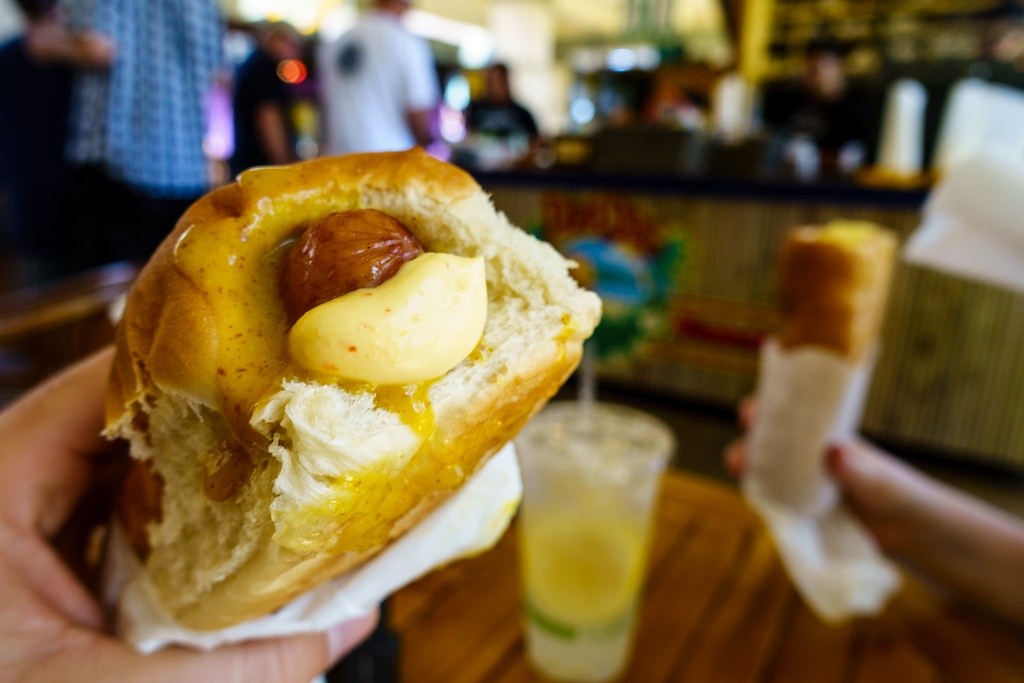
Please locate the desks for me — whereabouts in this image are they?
[364,467,1024,683]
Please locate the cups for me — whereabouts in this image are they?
[514,401,676,682]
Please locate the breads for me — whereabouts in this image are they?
[768,221,897,366]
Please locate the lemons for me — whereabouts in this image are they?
[519,513,646,626]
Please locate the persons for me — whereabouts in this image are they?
[722,388,1024,639]
[589,69,717,186]
[1,341,383,683]
[941,19,1024,101]
[779,34,877,188]
[313,0,443,158]
[28,0,224,273]
[233,21,303,167]
[459,62,540,143]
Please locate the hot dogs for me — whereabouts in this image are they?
[98,151,603,632]
